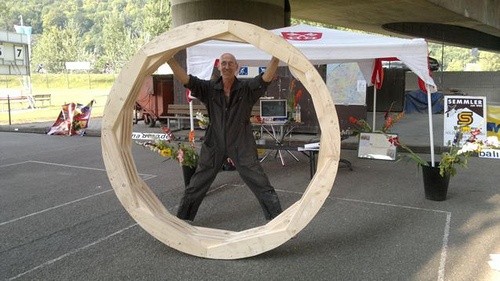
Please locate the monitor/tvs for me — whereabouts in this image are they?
[260,98,288,120]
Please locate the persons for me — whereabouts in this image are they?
[166,55,300,240]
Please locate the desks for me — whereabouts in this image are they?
[253,118,301,168]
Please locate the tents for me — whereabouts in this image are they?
[181,20,441,168]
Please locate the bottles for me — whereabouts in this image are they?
[296,103,302,123]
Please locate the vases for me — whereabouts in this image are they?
[141,124,202,189]
[385,121,484,202]
